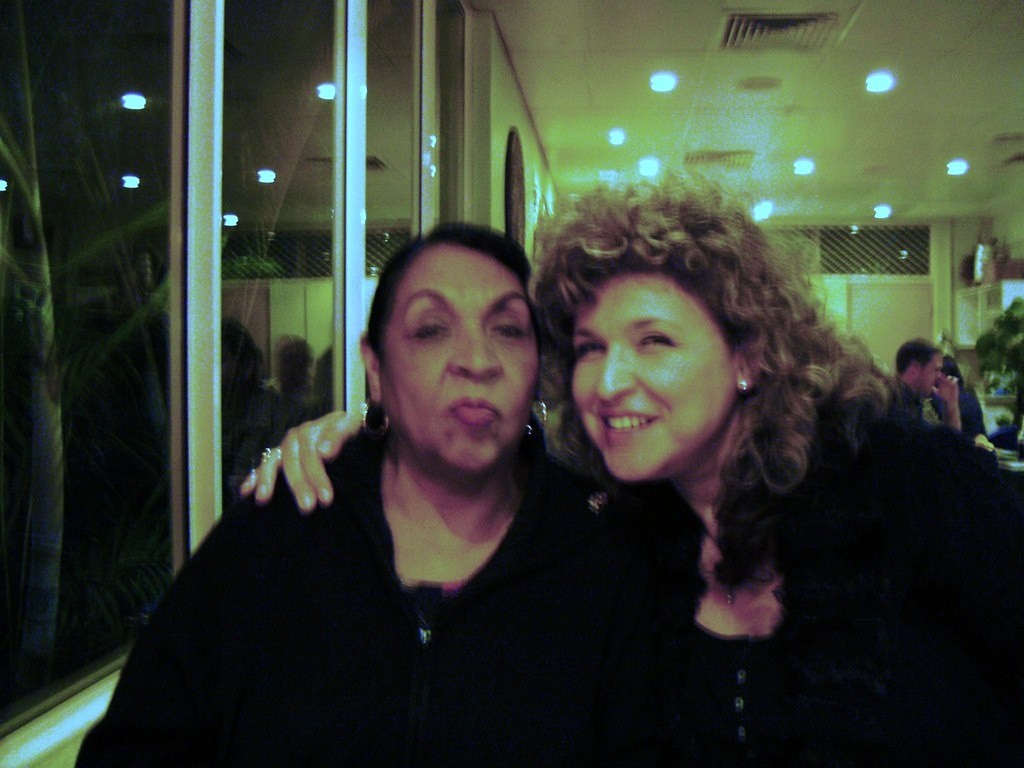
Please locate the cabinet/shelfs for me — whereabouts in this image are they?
[953,280,1023,350]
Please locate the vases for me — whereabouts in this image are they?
[975,243,995,283]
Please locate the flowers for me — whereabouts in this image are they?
[977,218,1000,245]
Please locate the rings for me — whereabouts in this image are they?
[262,446,283,463]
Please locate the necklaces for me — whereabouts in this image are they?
[709,530,742,605]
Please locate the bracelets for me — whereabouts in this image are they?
[360,402,369,427]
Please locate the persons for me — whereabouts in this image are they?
[74,189,1024,768]
